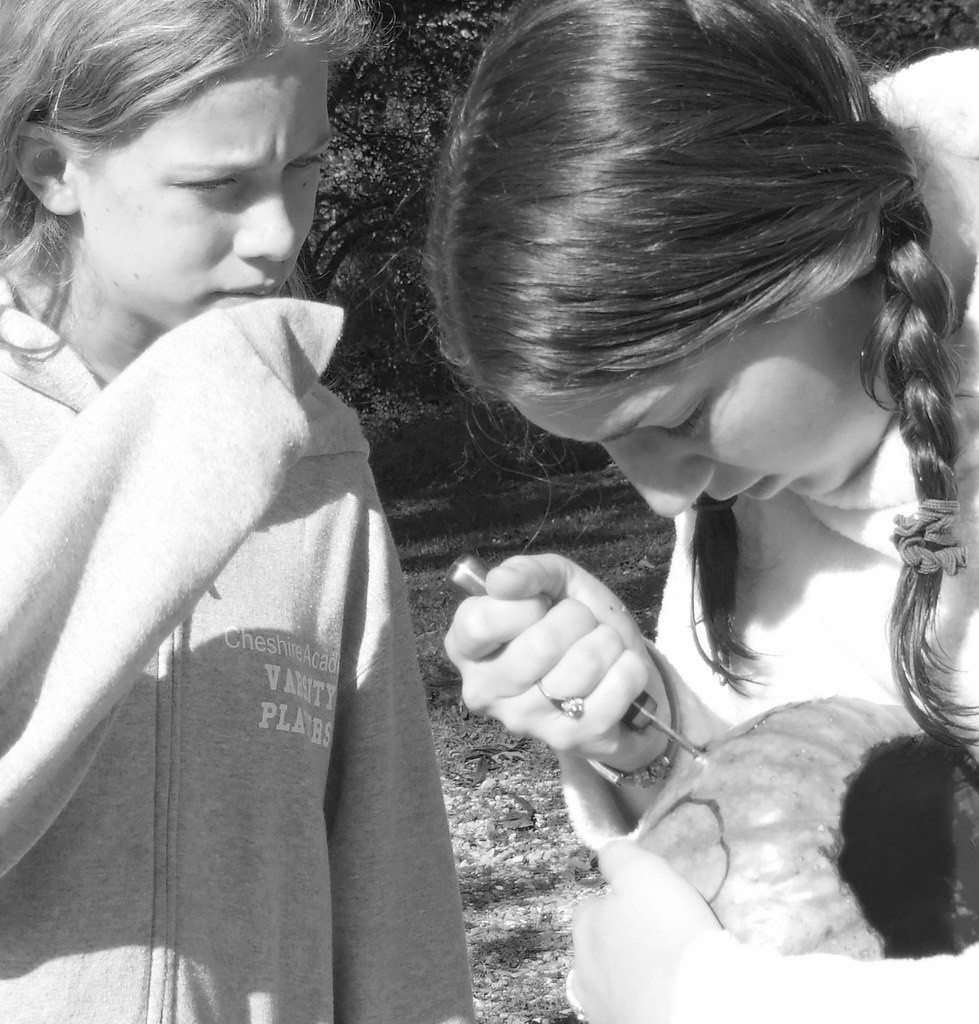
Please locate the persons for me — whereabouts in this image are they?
[0,1,476,1023]
[396,0,979,1023]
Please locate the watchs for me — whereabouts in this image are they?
[588,643,682,788]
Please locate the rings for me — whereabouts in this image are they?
[536,678,585,720]
